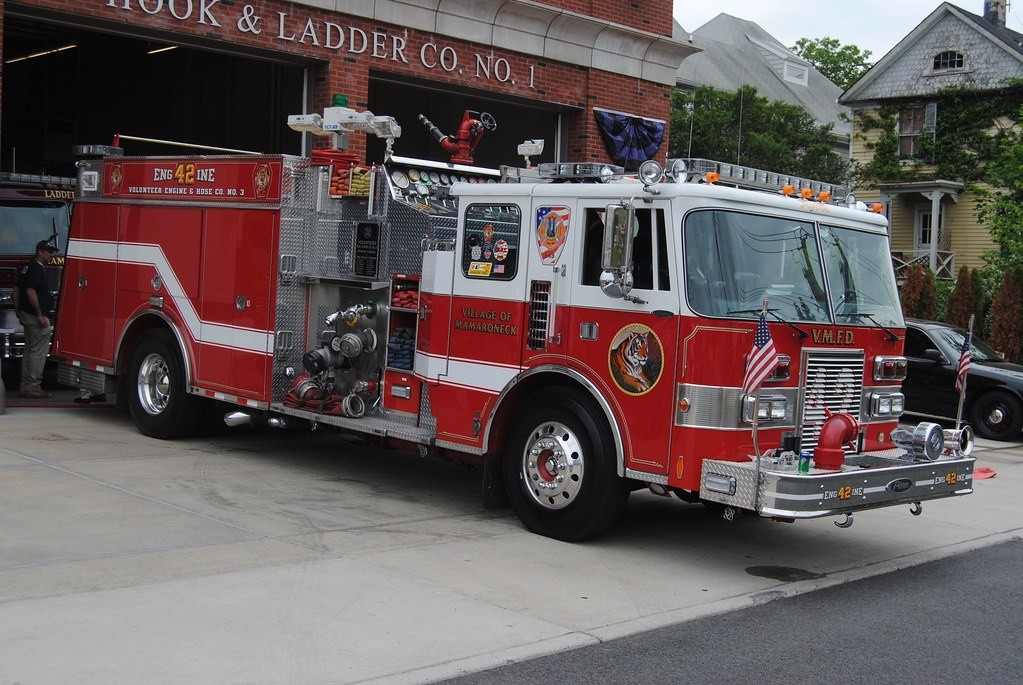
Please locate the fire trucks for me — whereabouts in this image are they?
[50,94,977,545]
[0,146,88,389]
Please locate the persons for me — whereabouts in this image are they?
[18,240,60,399]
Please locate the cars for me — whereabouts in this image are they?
[898,317,1023,441]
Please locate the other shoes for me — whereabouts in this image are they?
[20,389,53,399]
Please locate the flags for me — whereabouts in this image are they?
[742,313,781,394]
[954,328,971,401]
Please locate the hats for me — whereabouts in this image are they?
[36,240,59,253]
